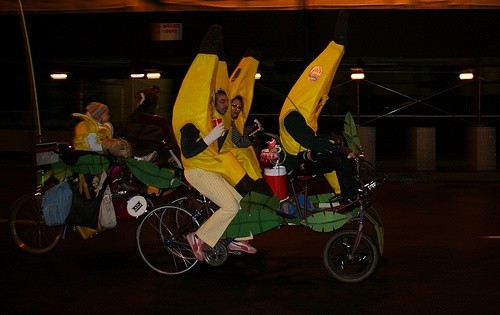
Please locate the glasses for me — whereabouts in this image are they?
[231,103,242,109]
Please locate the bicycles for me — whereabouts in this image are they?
[136,135,383,283]
[7,140,188,258]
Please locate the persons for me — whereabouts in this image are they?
[121,92,179,166]
[279,96,371,211]
[230,96,260,147]
[74,102,114,190]
[181,89,257,262]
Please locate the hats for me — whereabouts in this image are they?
[87,103,109,120]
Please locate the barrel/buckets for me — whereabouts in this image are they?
[264,166,288,200]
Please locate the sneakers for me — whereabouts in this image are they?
[187,233,205,261]
[228,240,258,254]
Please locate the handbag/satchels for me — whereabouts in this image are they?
[41,169,116,240]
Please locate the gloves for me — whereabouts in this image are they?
[203,122,225,146]
